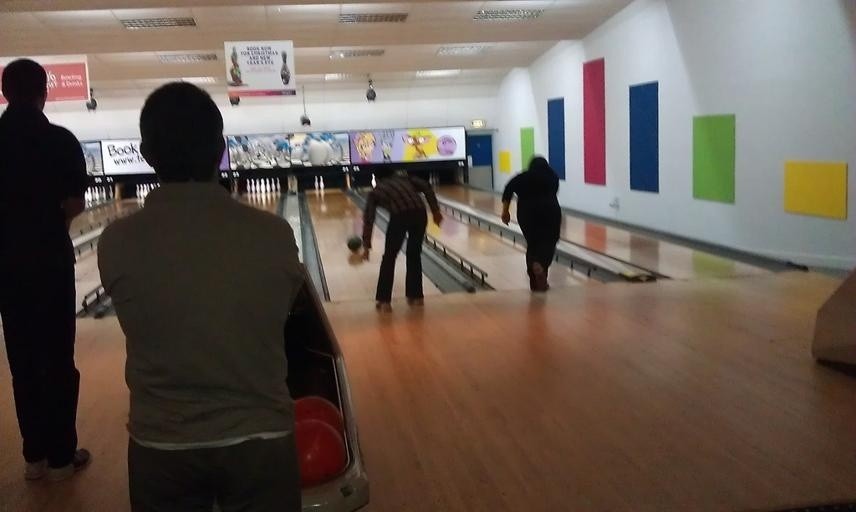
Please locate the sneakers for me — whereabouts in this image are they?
[375,301,392,311]
[407,297,424,305]
[47,448,90,484]
[24,459,47,481]
[533,262,547,289]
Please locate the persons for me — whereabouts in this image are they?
[499,157,562,293]
[359,164,444,314]
[0,58,94,485]
[96,77,309,510]
